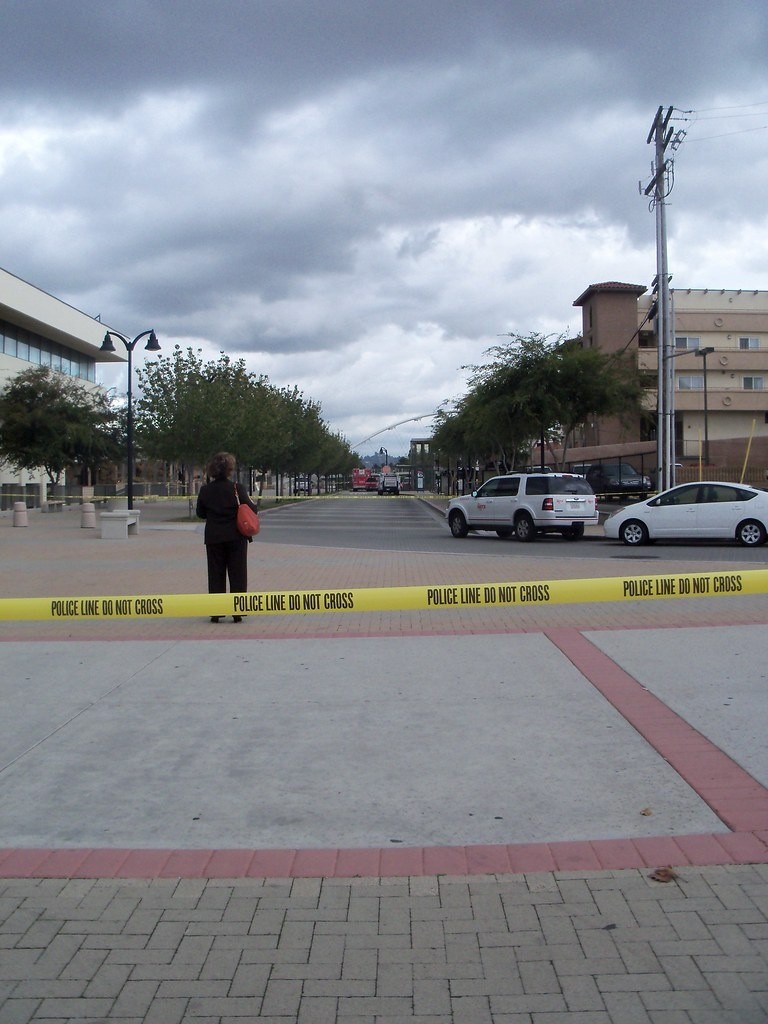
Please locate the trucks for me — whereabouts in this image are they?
[378,472,400,495]
[352,468,372,492]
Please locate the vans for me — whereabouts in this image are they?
[506,465,552,474]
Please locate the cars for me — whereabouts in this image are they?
[603,480,768,548]
[572,464,591,476]
[372,474,381,489]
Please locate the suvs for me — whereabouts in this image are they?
[587,464,651,501]
[293,477,312,494]
[445,470,600,541]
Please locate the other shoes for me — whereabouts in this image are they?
[211,617,219,623]
[235,615,242,623]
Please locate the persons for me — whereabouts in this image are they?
[196,452,257,623]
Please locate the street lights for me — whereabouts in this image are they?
[99,329,161,525]
[379,447,388,466]
[695,346,714,467]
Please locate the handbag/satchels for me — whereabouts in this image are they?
[234,481,260,537]
